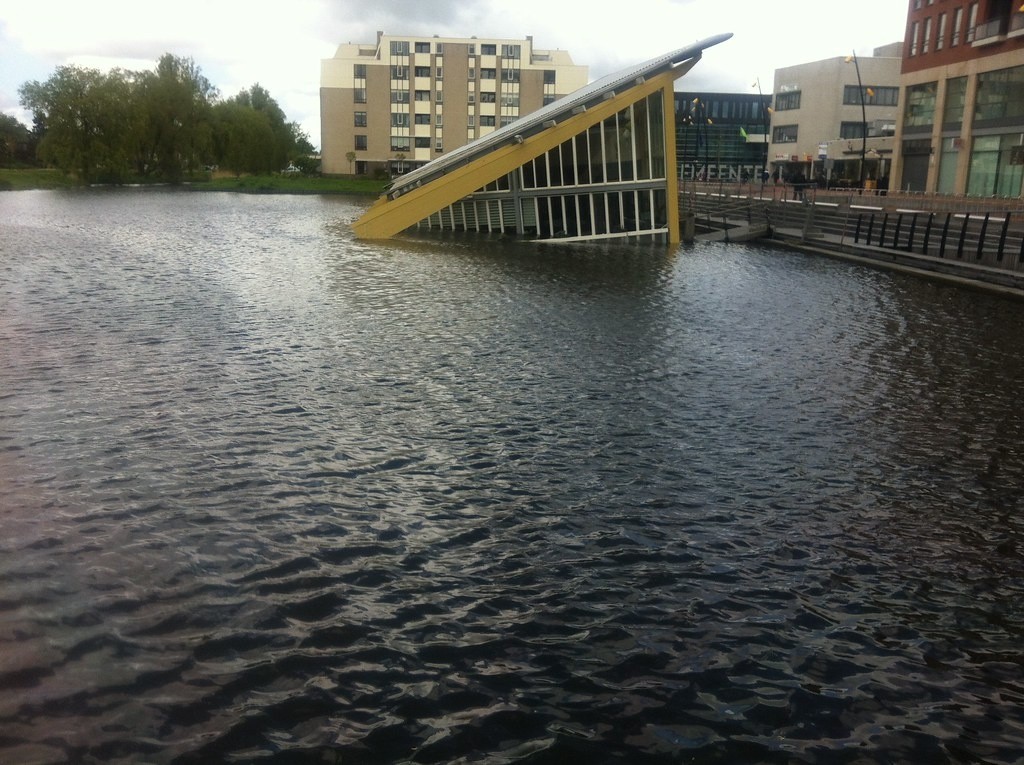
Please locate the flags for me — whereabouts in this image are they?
[739,128,749,140]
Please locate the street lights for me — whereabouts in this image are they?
[683,97,713,181]
[844,49,874,196]
[752,77,775,184]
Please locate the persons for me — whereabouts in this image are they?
[742,167,845,188]
[794,170,805,201]
